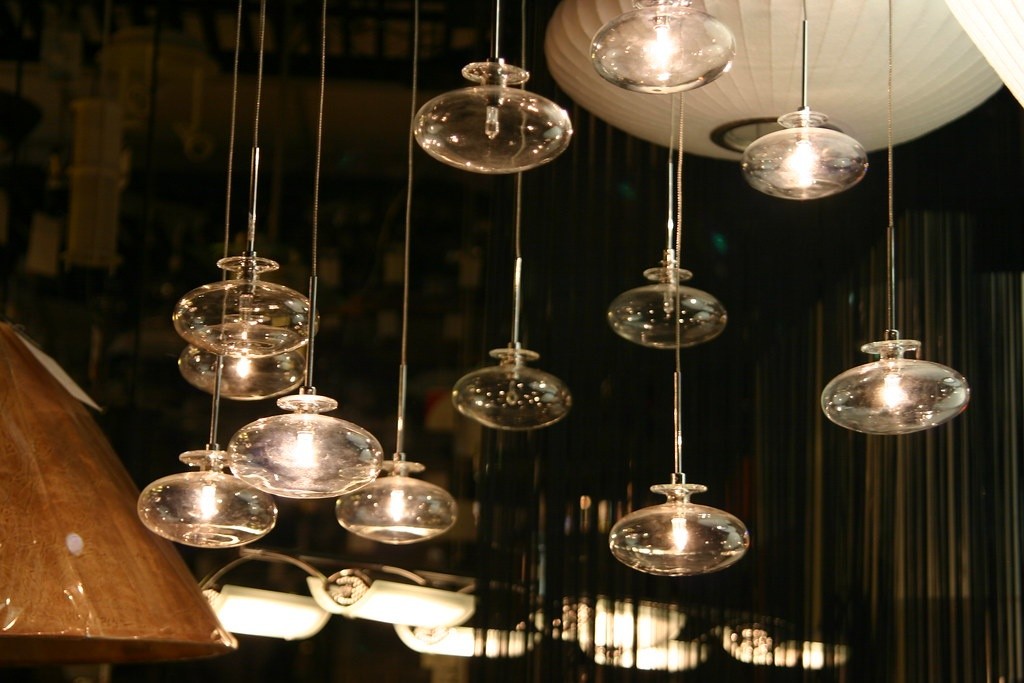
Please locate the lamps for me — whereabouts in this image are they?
[175,0,305,402]
[170,0,322,358]
[608,95,727,350]
[334,0,460,544]
[196,556,331,641]
[410,0,573,173]
[590,0,737,95]
[226,0,383,498]
[136,357,278,548]
[450,0,572,431]
[740,0,868,201]
[298,555,476,629]
[608,94,750,577]
[819,0,971,437]
